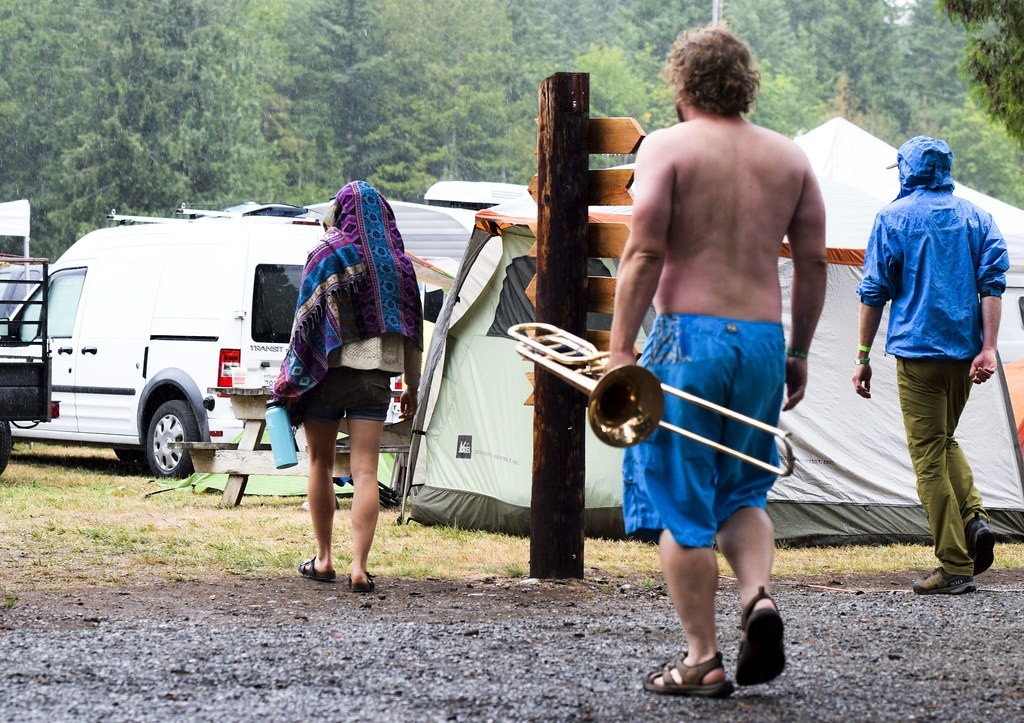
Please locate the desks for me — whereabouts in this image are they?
[207,387,404,508]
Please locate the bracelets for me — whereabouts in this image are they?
[854,358,870,364]
[858,345,871,352]
[787,348,808,358]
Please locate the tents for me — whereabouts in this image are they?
[411,117,1024,550]
[0,199,30,295]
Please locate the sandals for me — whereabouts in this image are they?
[735,587,786,684]
[642,650,734,697]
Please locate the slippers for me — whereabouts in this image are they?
[299,557,337,580]
[347,571,375,591]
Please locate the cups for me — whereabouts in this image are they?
[231,367,248,387]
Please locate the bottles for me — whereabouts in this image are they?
[265,400,299,469]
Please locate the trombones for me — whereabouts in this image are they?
[509,320,795,479]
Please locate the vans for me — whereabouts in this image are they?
[0,216,403,479]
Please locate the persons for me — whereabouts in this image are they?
[852,136,1009,594]
[275,180,424,591]
[603,26,827,694]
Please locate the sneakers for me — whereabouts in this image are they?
[912,564,977,596]
[965,511,995,577]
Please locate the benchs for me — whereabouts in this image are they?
[166,442,272,507]
[304,445,411,508]
